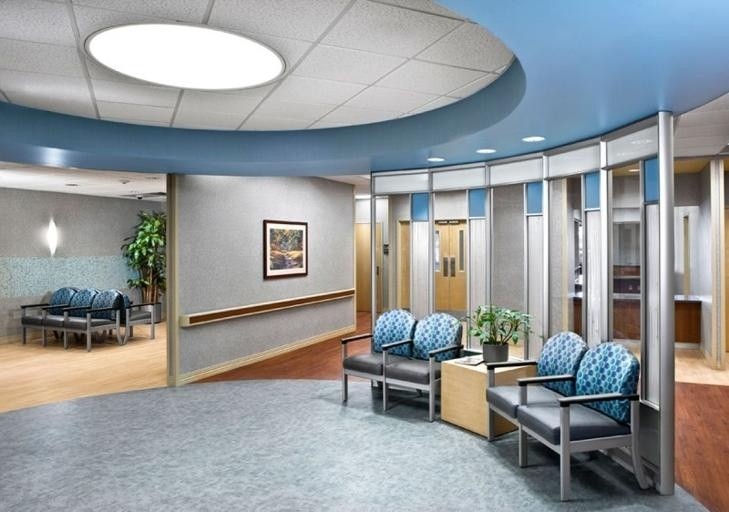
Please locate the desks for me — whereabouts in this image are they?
[440,355,534,438]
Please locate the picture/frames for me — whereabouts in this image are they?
[263,218,308,279]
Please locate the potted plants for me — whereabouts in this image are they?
[121,209,167,323]
[460,305,534,364]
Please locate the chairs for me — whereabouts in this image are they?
[486,332,589,467]
[339,309,416,411]
[518,342,650,500]
[21,286,155,350]
[378,311,464,422]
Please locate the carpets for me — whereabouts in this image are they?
[0,378,710,512]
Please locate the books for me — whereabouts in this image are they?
[456,356,484,366]
